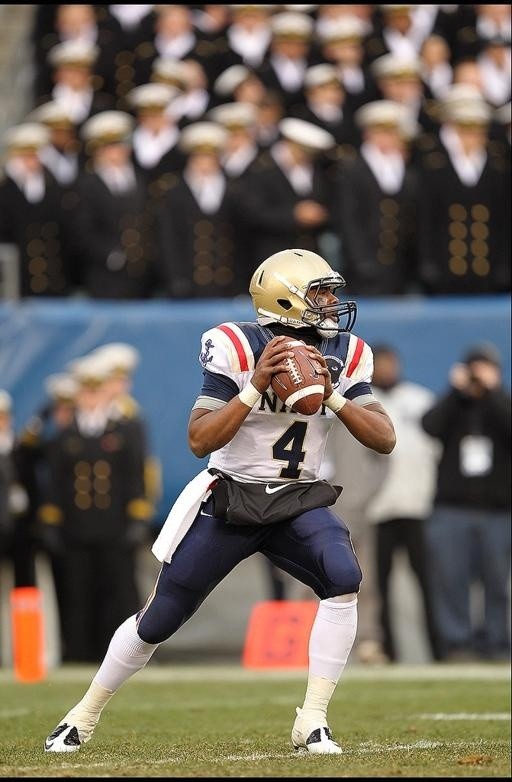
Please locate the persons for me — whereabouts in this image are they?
[279,341,511,663]
[41,248,397,756]
[0,3,512,305]
[1,342,157,665]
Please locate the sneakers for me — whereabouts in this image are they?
[290,707,342,756]
[44,707,94,753]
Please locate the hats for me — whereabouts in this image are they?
[0,0,512,152]
[46,341,136,400]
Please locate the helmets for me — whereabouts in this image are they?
[246,247,358,338]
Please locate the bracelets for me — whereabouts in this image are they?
[238,381,261,408]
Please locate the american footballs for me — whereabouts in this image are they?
[270,336,325,415]
